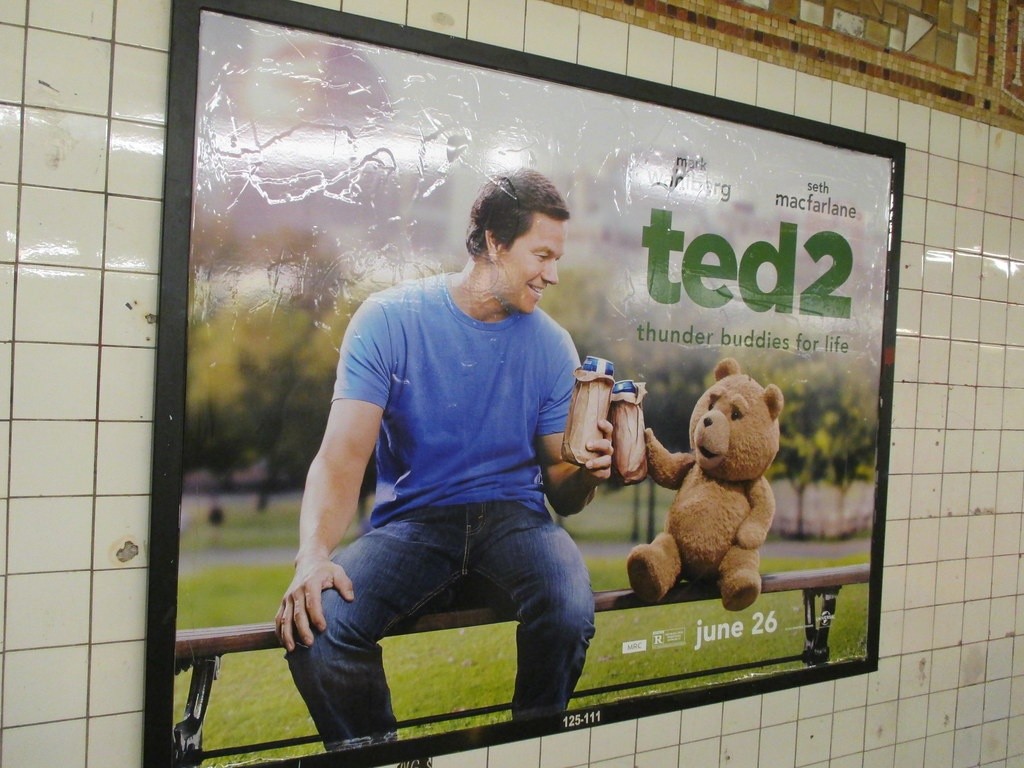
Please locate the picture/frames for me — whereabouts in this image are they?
[142,0,906,768]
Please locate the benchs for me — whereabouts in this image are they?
[169,563,872,766]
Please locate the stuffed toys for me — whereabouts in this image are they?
[627,358,783,613]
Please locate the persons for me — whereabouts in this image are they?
[275,168,613,753]
